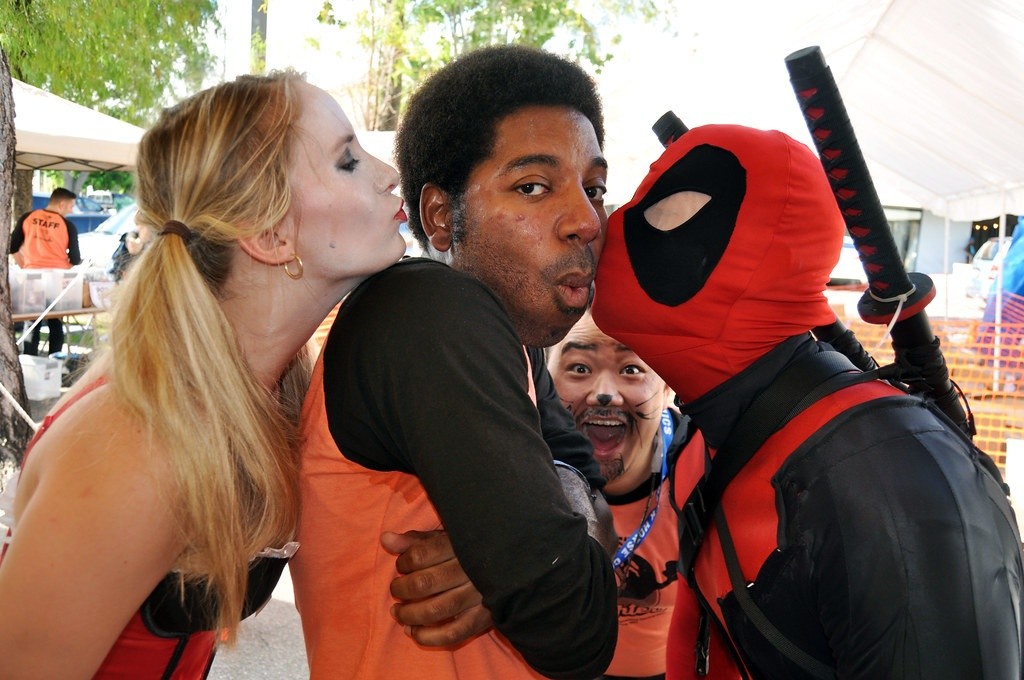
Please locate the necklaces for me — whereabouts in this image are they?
[626,435,657,578]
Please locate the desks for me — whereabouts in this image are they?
[10,303,108,377]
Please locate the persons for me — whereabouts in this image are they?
[2,72,409,679]
[593,125,1024,680]
[539,313,686,680]
[11,187,82,356]
[290,43,622,680]
[112,231,147,281]
[971,218,1024,390]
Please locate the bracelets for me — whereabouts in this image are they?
[554,458,598,502]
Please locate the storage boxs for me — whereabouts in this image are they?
[6,266,84,314]
[16,353,63,401]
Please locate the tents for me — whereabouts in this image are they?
[9,74,148,172]
